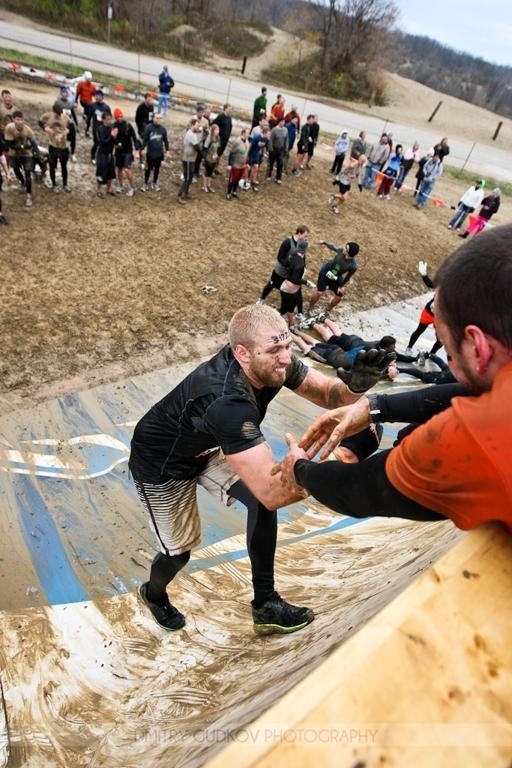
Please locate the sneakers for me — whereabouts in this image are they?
[20,172,72,206]
[328,193,335,207]
[286,308,330,332]
[204,184,216,193]
[252,594,314,635]
[227,191,238,200]
[333,203,340,214]
[256,297,266,304]
[292,163,314,176]
[97,182,161,198]
[176,191,192,204]
[243,177,282,192]
[419,349,426,366]
[137,582,185,630]
[404,347,412,356]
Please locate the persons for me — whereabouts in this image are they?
[227,86,319,200]
[1,89,52,207]
[257,224,459,385]
[158,66,174,119]
[38,70,111,195]
[270,224,509,543]
[448,179,501,239]
[330,131,449,214]
[97,93,169,197]
[127,301,395,634]
[176,103,233,205]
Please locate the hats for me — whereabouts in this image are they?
[346,242,359,257]
[84,71,92,79]
[94,91,103,96]
[114,108,123,119]
[60,87,68,92]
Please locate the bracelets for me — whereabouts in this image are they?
[366,391,382,424]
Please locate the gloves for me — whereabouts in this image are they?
[339,423,383,462]
[337,348,394,392]
[419,261,427,276]
